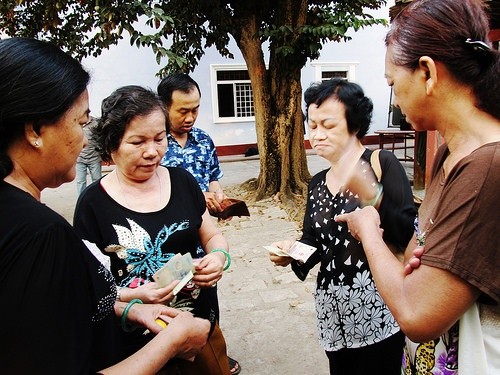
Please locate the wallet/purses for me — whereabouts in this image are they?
[211,198,250,218]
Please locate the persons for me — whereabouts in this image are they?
[73,86,231,375]
[157,74,242,375]
[268,78,418,375]
[0,37,210,375]
[334,0,500,375]
[75,116,102,197]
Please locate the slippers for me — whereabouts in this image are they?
[227,356,241,375]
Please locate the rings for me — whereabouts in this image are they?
[211,205,214,208]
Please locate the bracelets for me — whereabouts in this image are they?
[210,249,230,271]
[122,299,143,325]
[364,184,383,207]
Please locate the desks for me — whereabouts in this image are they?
[374,130,416,162]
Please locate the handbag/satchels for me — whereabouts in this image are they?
[370,149,423,209]
